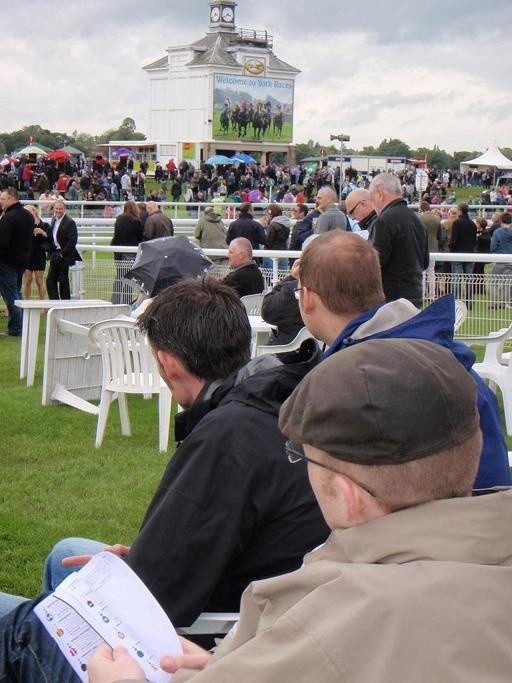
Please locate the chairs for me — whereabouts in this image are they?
[88,319,185,453]
[451,321,512,437]
[453,299,467,332]
[242,294,274,359]
[257,325,313,357]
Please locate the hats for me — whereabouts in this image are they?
[278,337,480,466]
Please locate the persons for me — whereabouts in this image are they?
[0,328,512,683]
[292,228,511,487]
[0,150,511,361]
[1,273,328,655]
[220,98,285,130]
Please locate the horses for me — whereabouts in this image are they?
[219,103,285,139]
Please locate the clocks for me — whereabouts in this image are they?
[222,7,234,23]
[210,7,220,22]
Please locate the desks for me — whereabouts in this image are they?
[42,303,132,416]
[14,299,113,388]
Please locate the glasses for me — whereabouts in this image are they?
[348,200,365,218]
[285,438,378,501]
[290,283,306,301]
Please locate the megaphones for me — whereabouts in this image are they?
[329,134,339,141]
